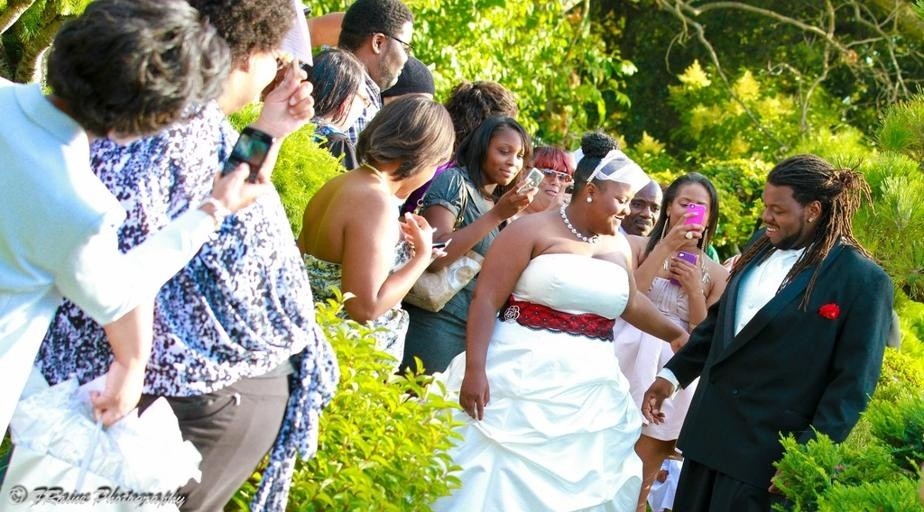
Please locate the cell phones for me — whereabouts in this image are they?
[684,203,706,239]
[221,127,274,184]
[671,252,697,285]
[516,167,544,194]
[565,185,575,193]
[432,242,445,248]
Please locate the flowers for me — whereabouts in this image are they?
[817,302,841,321]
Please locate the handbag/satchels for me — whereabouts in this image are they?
[0,445,182,511]
[402,250,484,313]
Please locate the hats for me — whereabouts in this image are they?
[382,56,434,95]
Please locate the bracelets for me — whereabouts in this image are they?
[194,197,230,222]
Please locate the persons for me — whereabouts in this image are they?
[0,1,270,511]
[296,2,726,512]
[32,0,340,510]
[641,152,895,512]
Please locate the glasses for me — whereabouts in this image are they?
[526,164,571,183]
